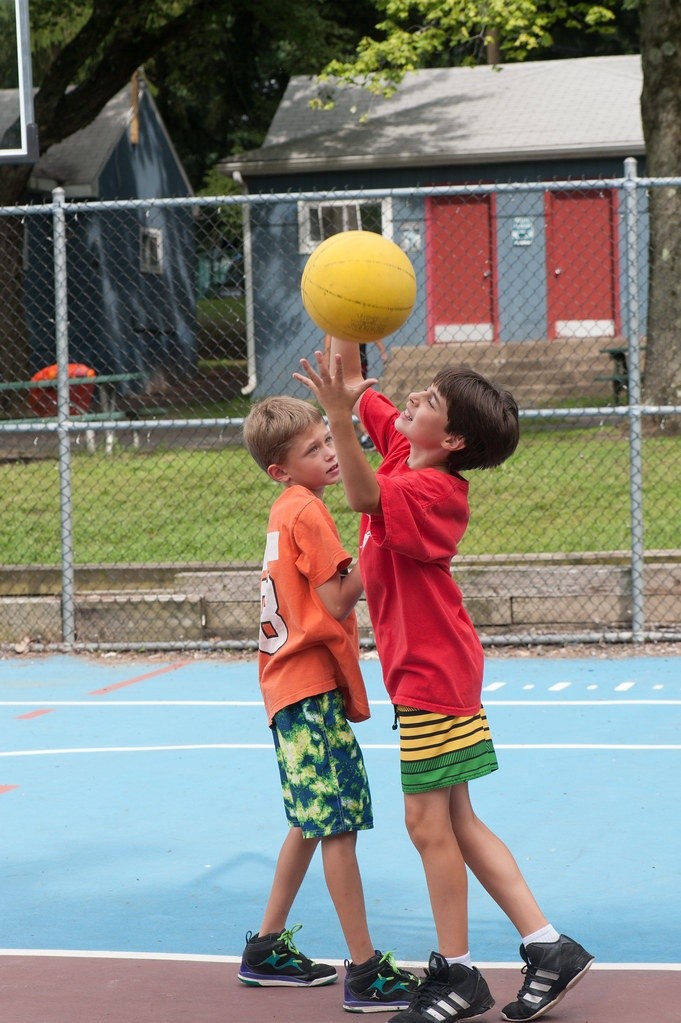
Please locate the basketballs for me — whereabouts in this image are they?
[299,231,416,341]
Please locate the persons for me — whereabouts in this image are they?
[238,395,428,1013]
[292,335,597,1023]
[325,332,387,453]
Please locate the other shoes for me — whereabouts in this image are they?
[358,434,376,453]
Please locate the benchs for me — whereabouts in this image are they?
[0,370,173,425]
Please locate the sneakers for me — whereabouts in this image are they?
[344,950,426,1013]
[386,951,495,1023]
[500,933,596,1021]
[237,924,338,987]
